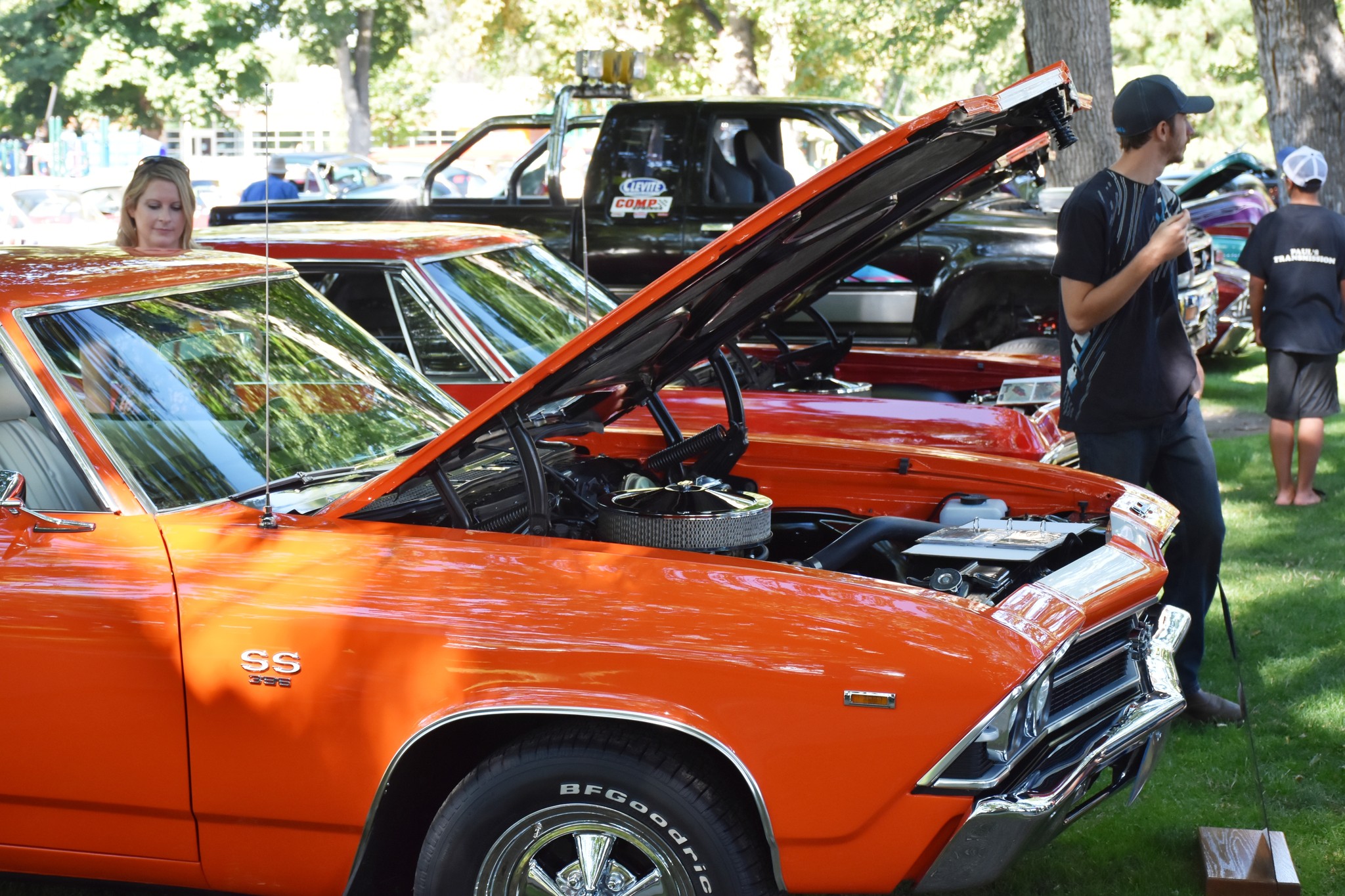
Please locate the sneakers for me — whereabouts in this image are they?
[1182,689,1241,723]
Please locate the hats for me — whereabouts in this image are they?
[1277,145,1327,188]
[1113,74,1214,138]
[267,156,287,174]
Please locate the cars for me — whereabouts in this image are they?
[0,64,1197,896]
[185,223,1090,472]
[0,137,507,246]
[1044,145,1287,366]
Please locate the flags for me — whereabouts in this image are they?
[0,117,162,175]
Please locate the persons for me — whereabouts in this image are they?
[240,155,299,201]
[1236,144,1345,505]
[82,157,241,415]
[1051,74,1243,722]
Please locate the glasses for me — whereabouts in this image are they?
[140,156,189,176]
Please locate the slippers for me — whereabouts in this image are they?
[1312,488,1326,500]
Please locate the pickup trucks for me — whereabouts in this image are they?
[208,95,1223,362]
